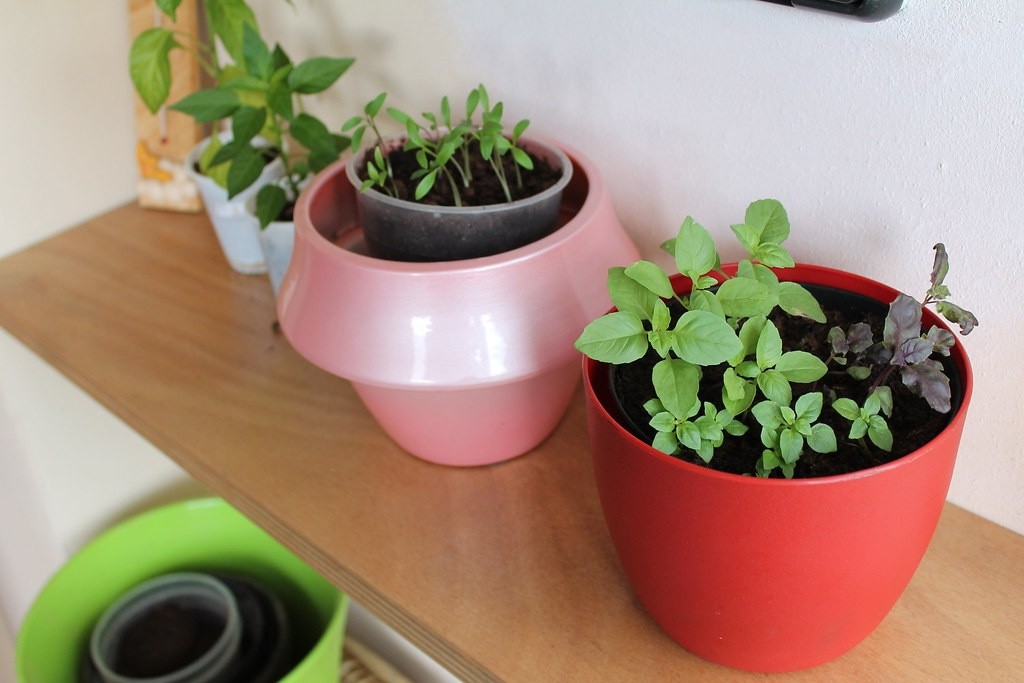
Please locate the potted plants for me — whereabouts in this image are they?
[161,25,357,299]
[339,83,575,264]
[128,0,299,275]
[572,197,979,674]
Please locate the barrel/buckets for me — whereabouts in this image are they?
[14,497,349,683]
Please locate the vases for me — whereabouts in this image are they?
[277,126,642,466]
[11,496,352,683]
